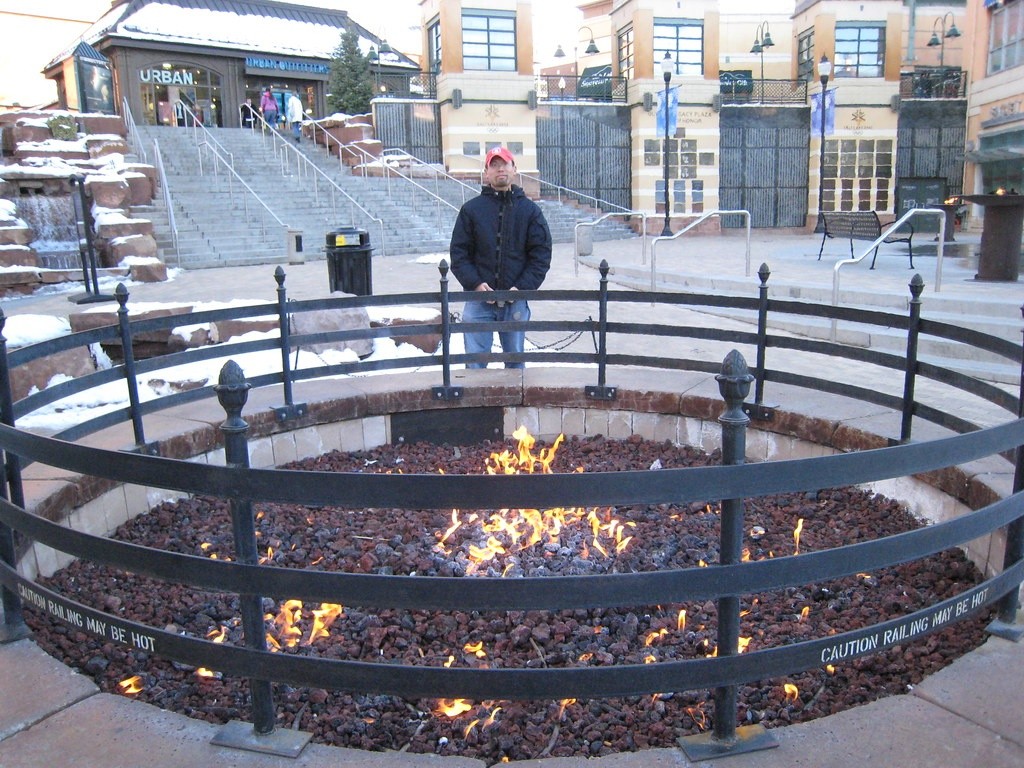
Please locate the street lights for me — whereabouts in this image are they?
[750,21,774,103]
[927,12,960,70]
[555,27,599,100]
[813,52,830,234]
[366,37,394,92]
[661,51,674,237]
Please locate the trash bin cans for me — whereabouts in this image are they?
[288,229,305,264]
[575,218,593,255]
[326,227,373,297]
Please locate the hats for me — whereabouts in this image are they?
[484,147,514,168]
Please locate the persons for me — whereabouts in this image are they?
[288,92,302,143]
[261,87,278,135]
[242,99,258,129]
[174,99,185,126]
[192,103,204,127]
[450,147,552,369]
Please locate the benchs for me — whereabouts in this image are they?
[817,210,914,269]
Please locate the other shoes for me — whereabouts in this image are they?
[296,136,301,143]
